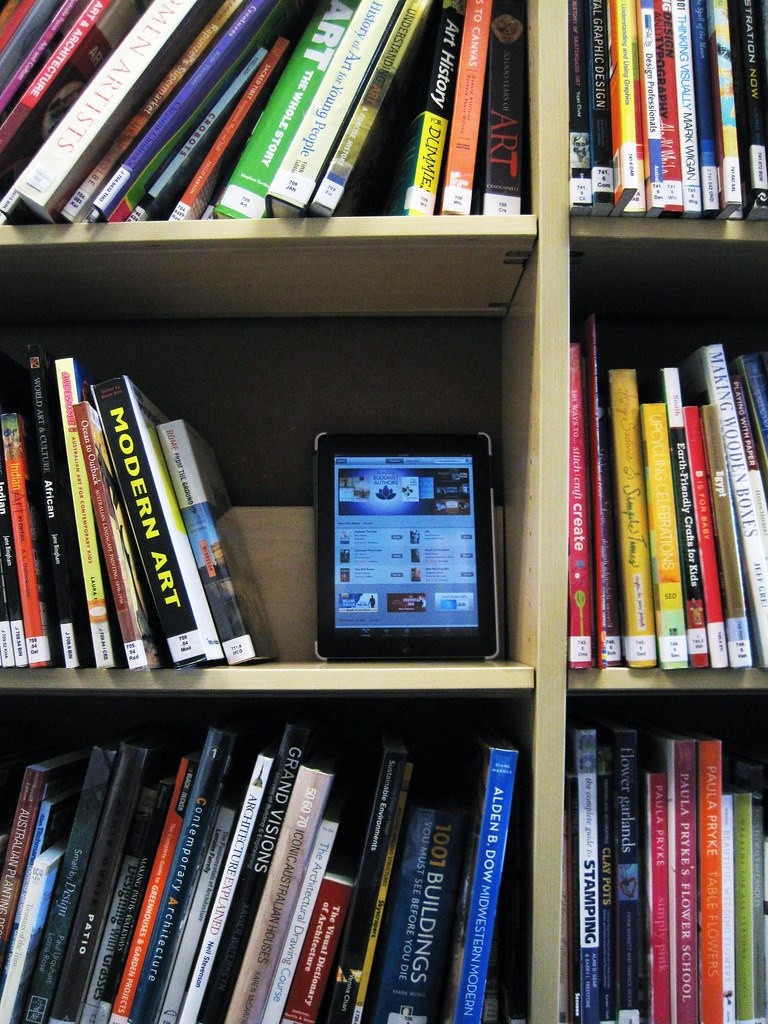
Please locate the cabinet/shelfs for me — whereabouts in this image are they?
[0,0,768,1024]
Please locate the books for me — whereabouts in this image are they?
[0,725,768,1024]
[0,313,768,675]
[0,0,768,221]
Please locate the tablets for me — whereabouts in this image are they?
[314,429,500,660]
